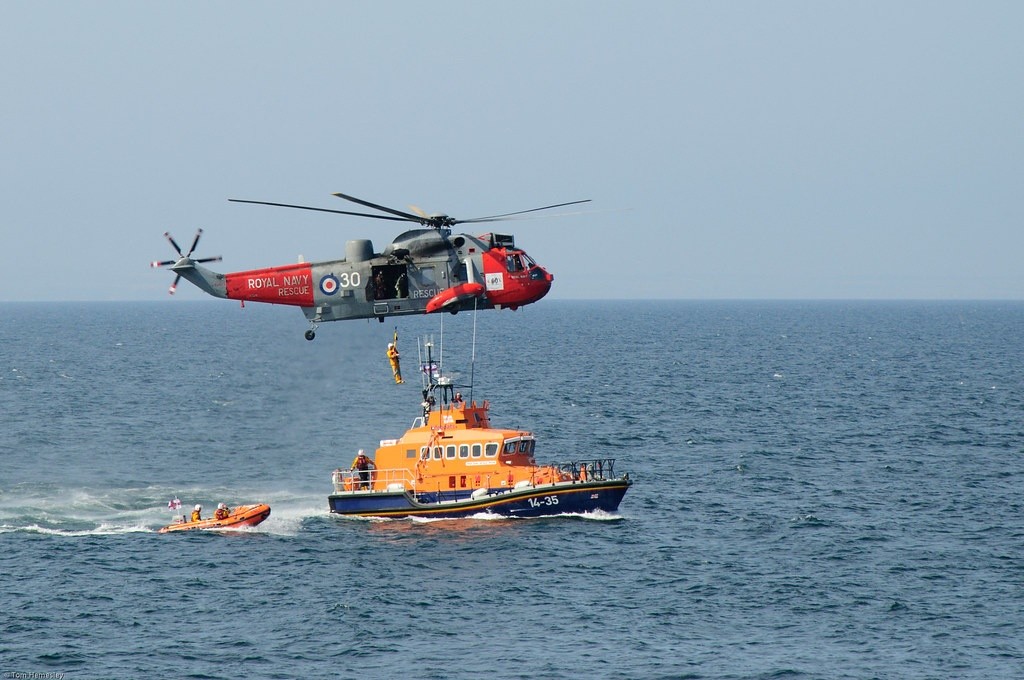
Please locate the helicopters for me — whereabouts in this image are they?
[146,190,593,342]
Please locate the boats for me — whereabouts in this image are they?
[156,494,272,534]
[328,295,634,522]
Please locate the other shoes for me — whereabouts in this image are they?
[396,380,405,384]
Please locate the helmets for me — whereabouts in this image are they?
[217,503,225,510]
[195,504,201,511]
[388,343,394,351]
[358,449,365,456]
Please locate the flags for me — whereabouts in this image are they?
[168,499,181,512]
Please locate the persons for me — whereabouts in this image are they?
[350,450,376,490]
[374,270,387,299]
[395,273,408,297]
[387,343,405,384]
[215,503,231,521]
[450,393,464,410]
[191,504,202,522]
